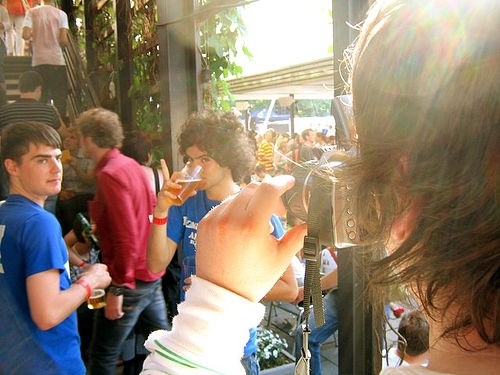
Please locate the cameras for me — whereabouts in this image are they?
[281,95,388,247]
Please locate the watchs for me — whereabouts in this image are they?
[108,285,124,296]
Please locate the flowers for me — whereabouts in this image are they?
[255,326,288,369]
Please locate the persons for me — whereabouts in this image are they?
[0,0,500,375]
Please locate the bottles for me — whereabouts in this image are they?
[77,213,100,251]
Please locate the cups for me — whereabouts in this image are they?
[74,260,106,310]
[167,160,204,206]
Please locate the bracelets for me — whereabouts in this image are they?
[75,280,92,301]
[78,261,86,268]
[148,214,168,225]
